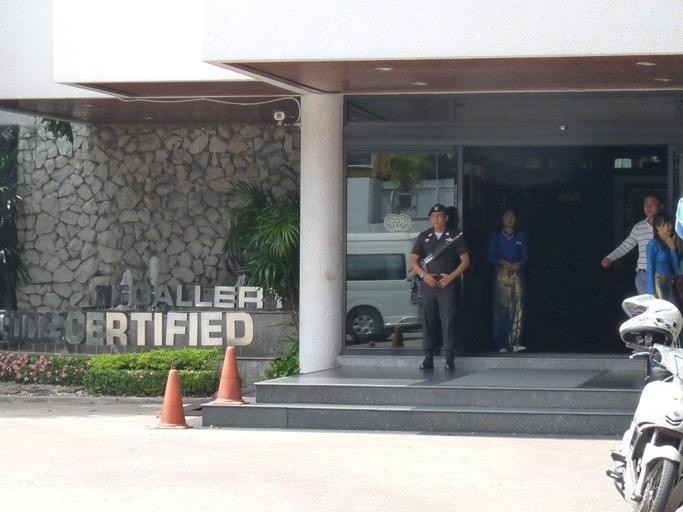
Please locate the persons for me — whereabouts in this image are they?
[643,208,682,347]
[598,187,664,359]
[485,206,532,354]
[408,203,471,375]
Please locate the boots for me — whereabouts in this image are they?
[419,353,455,369]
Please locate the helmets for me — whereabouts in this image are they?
[620,292,683,347]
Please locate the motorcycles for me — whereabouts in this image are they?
[606,294,683,512]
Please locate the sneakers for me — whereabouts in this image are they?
[497,344,527,352]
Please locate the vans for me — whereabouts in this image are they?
[346,231,422,343]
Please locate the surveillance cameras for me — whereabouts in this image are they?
[274,111,287,121]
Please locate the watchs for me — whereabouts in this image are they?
[417,270,427,279]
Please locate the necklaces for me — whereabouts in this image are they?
[500,228,516,240]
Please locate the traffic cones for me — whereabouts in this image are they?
[210,345,250,406]
[149,369,194,430]
[390,326,405,349]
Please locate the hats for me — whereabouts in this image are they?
[429,203,448,216]
